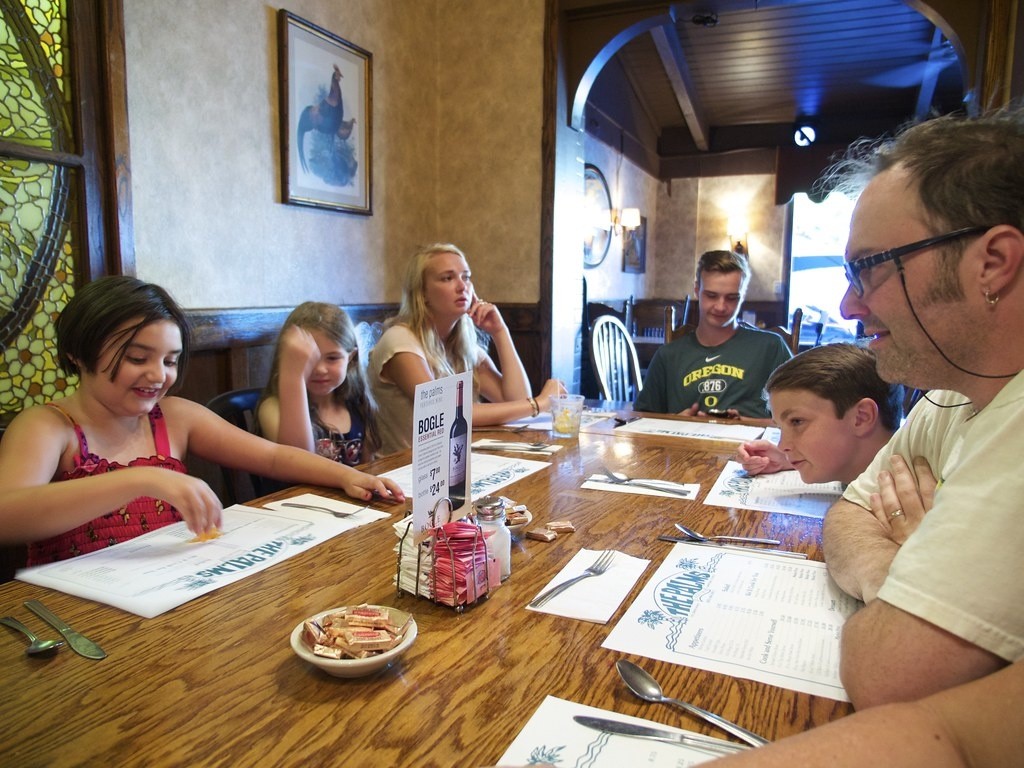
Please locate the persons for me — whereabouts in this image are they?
[737,342,899,486]
[820,107,1024,714]
[250,301,380,494]
[697,656,1024,768]
[1,275,406,569]
[632,251,794,422]
[368,244,569,461]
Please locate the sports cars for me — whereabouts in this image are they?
[789,304,854,341]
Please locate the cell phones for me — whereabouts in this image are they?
[707,409,729,418]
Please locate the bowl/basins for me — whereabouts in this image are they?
[290,605,418,678]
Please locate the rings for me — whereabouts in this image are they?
[888,510,904,522]
[480,302,484,305]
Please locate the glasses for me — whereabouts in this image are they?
[842,221,995,299]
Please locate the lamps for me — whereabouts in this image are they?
[621,207,645,227]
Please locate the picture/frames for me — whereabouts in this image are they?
[625,213,651,273]
[276,7,375,215]
[586,161,618,270]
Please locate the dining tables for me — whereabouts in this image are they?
[0,409,870,768]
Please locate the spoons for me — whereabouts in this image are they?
[0,615,63,655]
[615,416,639,423]
[674,523,781,546]
[616,659,771,749]
[471,425,528,432]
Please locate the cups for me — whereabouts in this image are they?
[548,394,584,438]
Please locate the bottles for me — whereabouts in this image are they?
[474,495,506,529]
[450,381,467,512]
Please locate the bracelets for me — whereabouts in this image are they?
[534,399,539,415]
[527,398,538,416]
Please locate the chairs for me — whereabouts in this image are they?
[583,277,803,404]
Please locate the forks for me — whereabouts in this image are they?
[282,502,369,517]
[598,466,687,497]
[528,549,616,608]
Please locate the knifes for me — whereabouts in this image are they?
[23,599,106,659]
[573,716,753,756]
[657,535,809,560]
